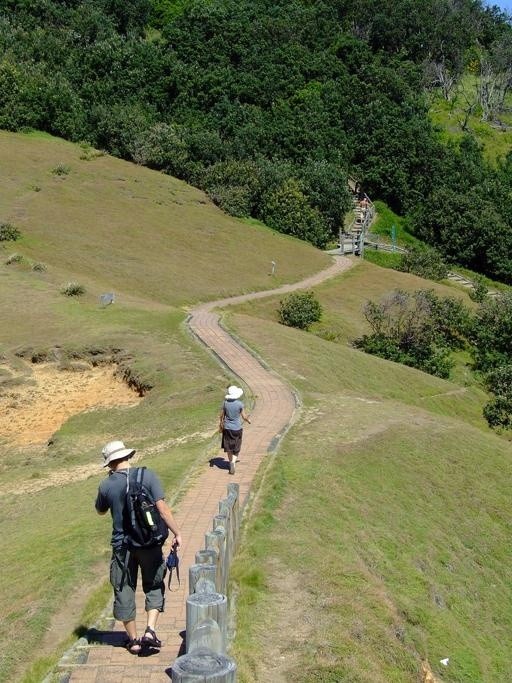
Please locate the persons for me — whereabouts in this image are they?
[359,198,371,223]
[220,385,251,474]
[95,440,181,656]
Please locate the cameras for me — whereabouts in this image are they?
[166,553,179,569]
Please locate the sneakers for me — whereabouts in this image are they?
[229,461,235,474]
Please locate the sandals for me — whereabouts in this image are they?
[142,628,164,649]
[125,639,142,655]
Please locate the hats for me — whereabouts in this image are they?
[102,438,137,469]
[224,385,243,400]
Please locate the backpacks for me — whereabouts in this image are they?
[127,465,167,549]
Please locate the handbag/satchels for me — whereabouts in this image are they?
[166,552,178,568]
[218,420,225,434]
